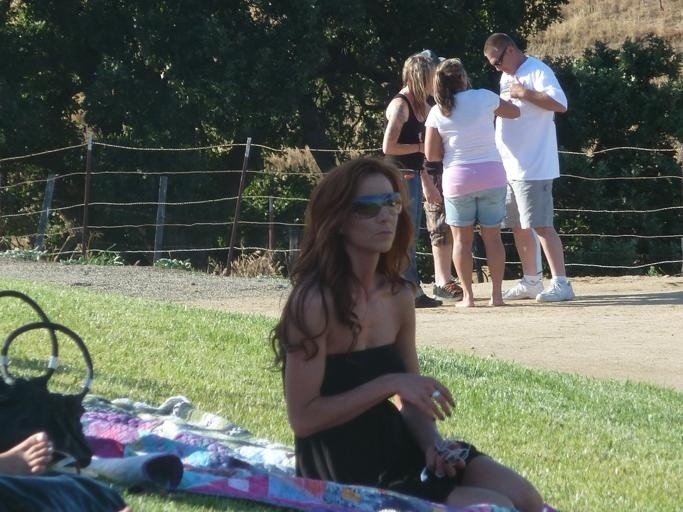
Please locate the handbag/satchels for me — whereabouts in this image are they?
[0,381,91,469]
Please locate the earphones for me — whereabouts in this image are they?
[419,466,429,482]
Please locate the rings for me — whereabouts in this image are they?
[432,391,441,398]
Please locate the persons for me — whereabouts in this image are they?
[423,57,520,308]
[382,48,439,310]
[484,30,576,302]
[267,155,546,511]
[0,432,52,480]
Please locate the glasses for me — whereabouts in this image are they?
[353,191,403,220]
[495,46,508,67]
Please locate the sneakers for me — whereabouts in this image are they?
[502,277,544,299]
[414,295,443,308]
[433,282,463,301]
[536,279,576,302]
